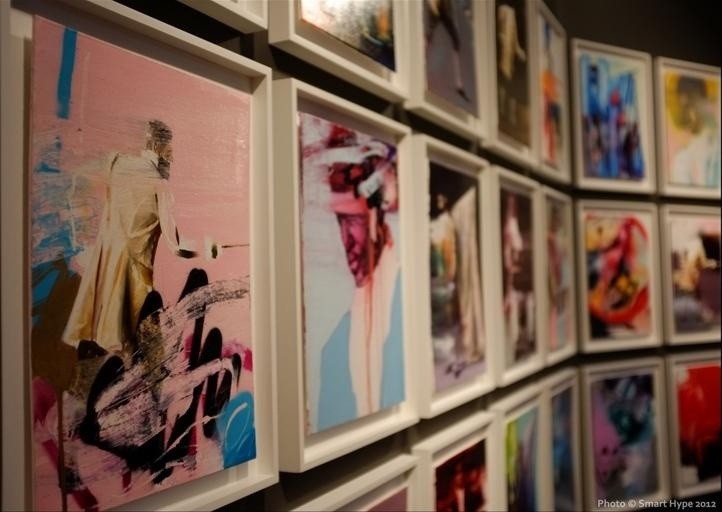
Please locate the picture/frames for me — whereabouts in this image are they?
[0,1,722,511]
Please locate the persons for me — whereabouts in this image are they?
[58,0,722,489]
